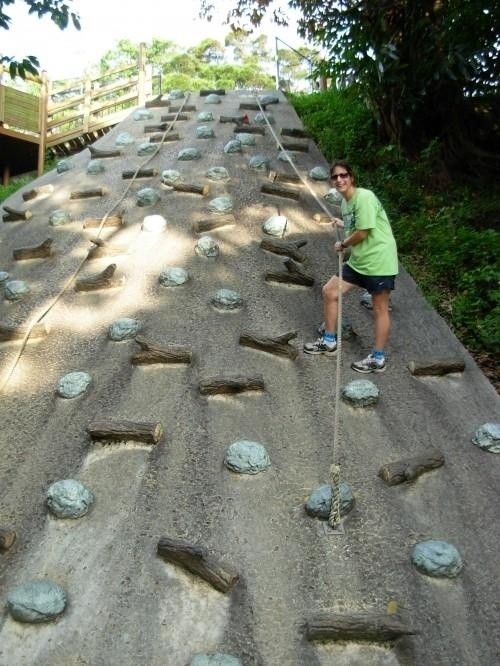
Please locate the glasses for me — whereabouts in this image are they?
[331,173,349,180]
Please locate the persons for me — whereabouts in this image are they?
[303,161,400,375]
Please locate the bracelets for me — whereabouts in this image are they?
[341,239,347,249]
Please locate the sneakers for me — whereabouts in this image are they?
[350,355,386,374]
[303,337,338,357]
